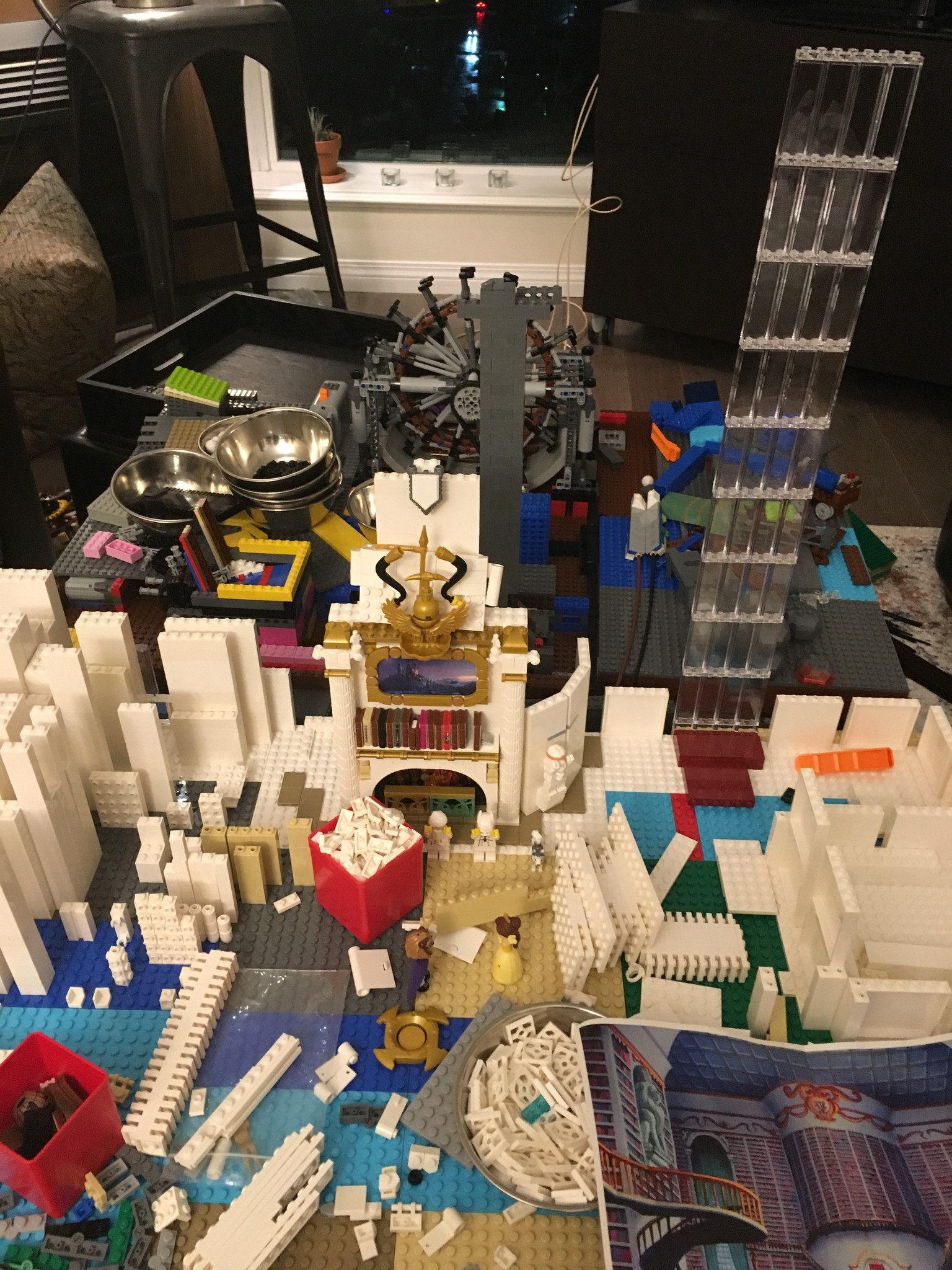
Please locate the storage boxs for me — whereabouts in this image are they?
[77,289,396,460]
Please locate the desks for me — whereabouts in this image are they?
[249,159,594,301]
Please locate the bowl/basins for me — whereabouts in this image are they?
[455,1001,611,1212]
[198,407,344,534]
[110,448,226,535]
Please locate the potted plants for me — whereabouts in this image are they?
[305,106,347,184]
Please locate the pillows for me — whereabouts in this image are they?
[0,161,119,464]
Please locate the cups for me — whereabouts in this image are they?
[488,169,509,188]
[434,168,455,187]
[381,168,401,187]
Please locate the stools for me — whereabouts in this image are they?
[53,0,343,320]
